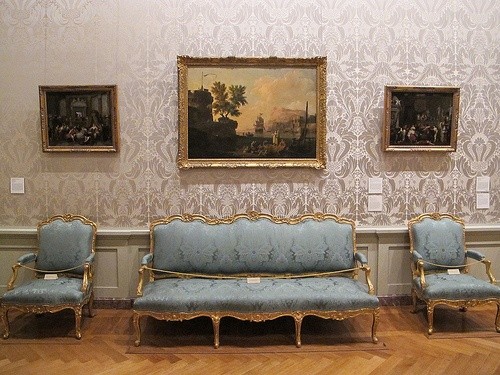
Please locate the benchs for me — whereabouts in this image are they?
[132,210,381,349]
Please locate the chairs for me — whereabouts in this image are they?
[407,212,500,335]
[0,214,97,340]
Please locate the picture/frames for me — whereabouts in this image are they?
[382,85,460,153]
[39,84,119,153]
[176,53,327,171]
